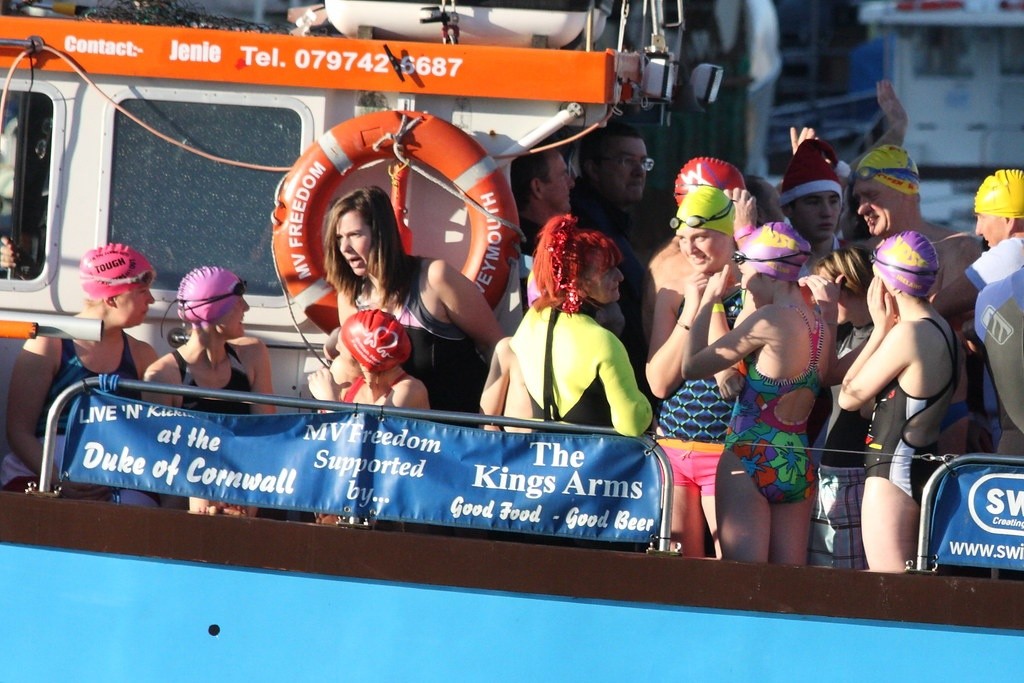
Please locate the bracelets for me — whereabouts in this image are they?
[675,320,691,331]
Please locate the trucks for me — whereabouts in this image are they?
[859,0,1023,250]
[1,0,723,522]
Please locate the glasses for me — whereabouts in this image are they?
[669,215,704,230]
[731,251,747,263]
[594,154,654,172]
[850,167,884,183]
[867,247,880,262]
[232,280,248,296]
[135,270,158,288]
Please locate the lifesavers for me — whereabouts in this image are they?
[268,104,523,371]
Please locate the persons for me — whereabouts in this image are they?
[508,212,652,439]
[306,306,432,524]
[140,266,281,517]
[321,186,503,417]
[0,241,158,504]
[643,185,743,558]
[931,166,1024,461]
[475,78,1024,577]
[0,234,21,270]
[680,221,832,566]
[838,230,959,573]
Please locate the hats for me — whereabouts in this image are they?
[738,221,812,280]
[340,308,412,374]
[77,242,155,300]
[871,231,939,296]
[779,138,849,207]
[177,266,242,327]
[974,169,1024,219]
[675,187,736,237]
[855,144,920,200]
[672,157,746,208]
